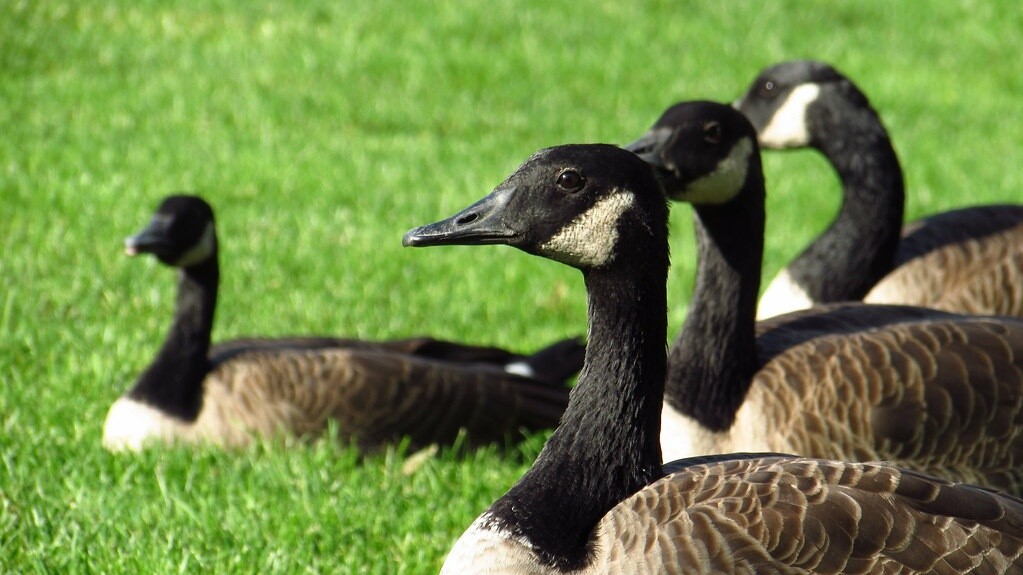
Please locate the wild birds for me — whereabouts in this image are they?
[624,100,1023,500]
[402,144,1023,575]
[99,192,585,456]
[730,60,1023,321]
